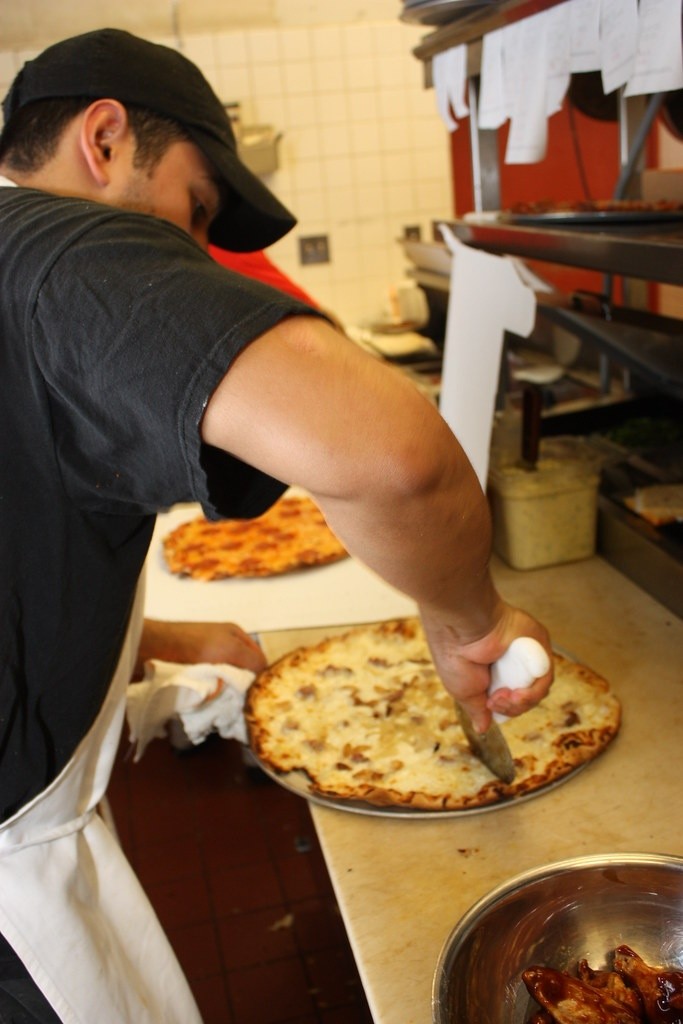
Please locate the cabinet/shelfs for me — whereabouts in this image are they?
[396,0,683,620]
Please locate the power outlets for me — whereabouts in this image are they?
[404,226,420,239]
[301,236,328,263]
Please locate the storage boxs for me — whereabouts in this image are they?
[637,168,683,206]
[487,459,601,570]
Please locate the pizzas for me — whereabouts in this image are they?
[162,495,348,578]
[243,617,622,810]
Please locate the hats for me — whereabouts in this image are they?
[0,27,298,254]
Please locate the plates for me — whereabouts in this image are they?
[249,643,591,819]
[510,210,683,225]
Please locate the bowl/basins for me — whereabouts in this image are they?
[431,851,683,1024]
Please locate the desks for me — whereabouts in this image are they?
[257,550,683,1024]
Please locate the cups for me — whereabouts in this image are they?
[491,434,610,571]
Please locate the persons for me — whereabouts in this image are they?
[0,27,555,1024]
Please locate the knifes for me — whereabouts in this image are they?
[453,636,551,783]
